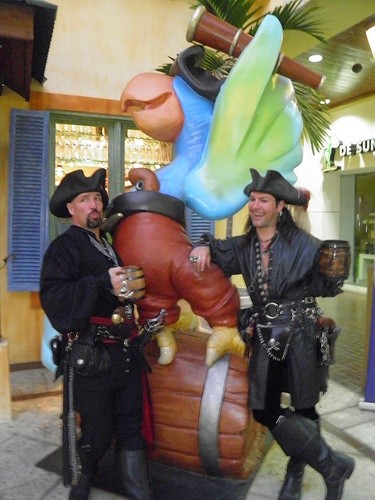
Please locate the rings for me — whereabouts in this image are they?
[189,256,197,262]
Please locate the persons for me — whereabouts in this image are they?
[39,169,153,500]
[189,168,356,500]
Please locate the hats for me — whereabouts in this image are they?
[50,168,109,218]
[244,168,298,203]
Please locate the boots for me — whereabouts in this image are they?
[278,418,320,500]
[273,407,355,500]
[114,443,154,500]
[69,441,103,500]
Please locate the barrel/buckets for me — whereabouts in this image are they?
[140,330,268,481]
[121,264,146,300]
[319,240,351,281]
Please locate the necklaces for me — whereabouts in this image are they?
[247,234,273,293]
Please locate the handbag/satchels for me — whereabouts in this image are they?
[69,334,111,376]
